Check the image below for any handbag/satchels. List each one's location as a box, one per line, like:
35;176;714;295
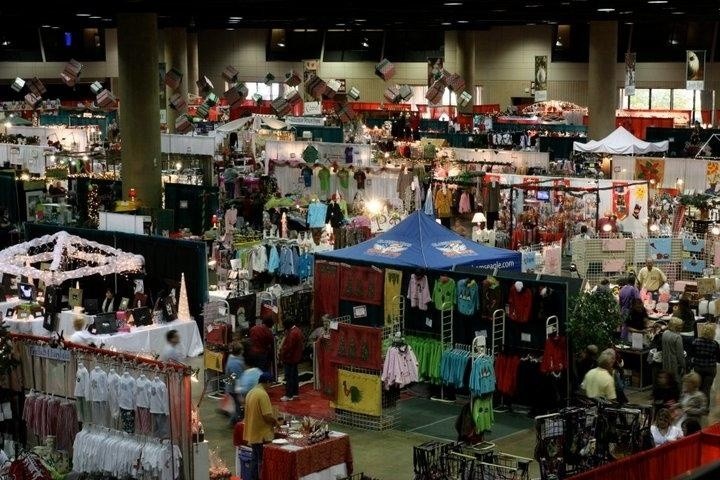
533;396;655;480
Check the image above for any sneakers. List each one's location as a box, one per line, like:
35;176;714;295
268;381;300;402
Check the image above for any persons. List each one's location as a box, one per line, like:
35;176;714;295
73;364;169;438
222;160;241;202
52;181;67;197
97;288;119;314
577;257;720;455
69;316;95;346
160;314;330;480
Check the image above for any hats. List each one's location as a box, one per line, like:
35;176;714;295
260;372;277;382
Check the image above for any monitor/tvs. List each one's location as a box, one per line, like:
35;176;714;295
536;190;550;201
353;305;368;319
17;282;37;301
